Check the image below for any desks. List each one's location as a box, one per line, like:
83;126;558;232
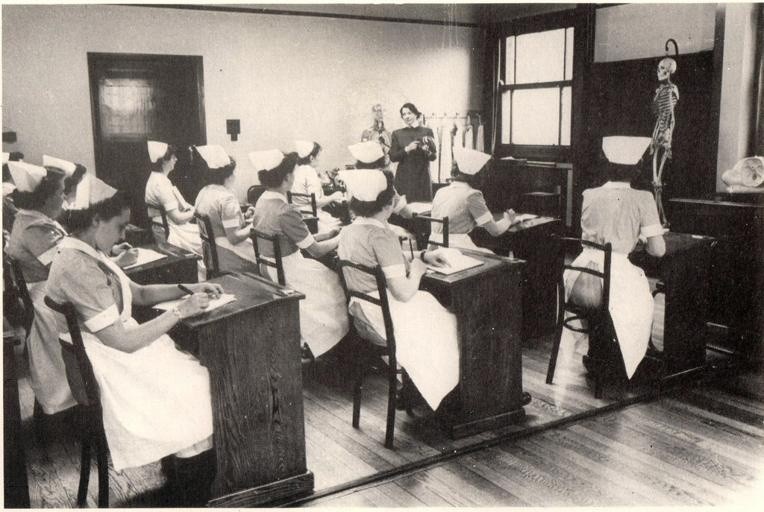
489;159;571;220
634;231;716;392
305;218;320;232
420;248;528;441
161;270;315;507
3;314;30;508
479;210;563;341
120;242;202;285
125;224;146;245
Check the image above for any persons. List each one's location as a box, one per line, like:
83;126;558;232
557;135;667;386
191;145;258;277
388;104;437;202
347;141;411;224
289;139;343;233
427;148;515;257
334;167;532;412
3;139;224;488
247;149;352;360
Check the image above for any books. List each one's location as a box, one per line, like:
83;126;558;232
423;247;485;275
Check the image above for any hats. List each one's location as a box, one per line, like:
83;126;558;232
247;149;285;172
2;152;10;166
348;139;385;164
42;154;77;179
452;146;492;176
195;144;232;170
296;140;315;159
146;139;169;164
74;174;119;211
7;160;48;194
601;134;653;166
337;168;390;202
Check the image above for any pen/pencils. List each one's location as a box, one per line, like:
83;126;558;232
123;245;131;250
409;239;414;258
178;285;194;295
505;208;509;214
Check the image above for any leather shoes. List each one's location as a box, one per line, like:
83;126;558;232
522;391;532;406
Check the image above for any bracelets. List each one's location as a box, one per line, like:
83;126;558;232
420;250;427;263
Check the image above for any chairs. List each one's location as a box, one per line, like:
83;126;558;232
193;213;227;279
3;251;35;357
247;227;328;388
546;231;617;399
522;185;562;218
287;191;317;218
43;294;110;508
142;201;169;242
333;251;424;450
412;212;449;249
